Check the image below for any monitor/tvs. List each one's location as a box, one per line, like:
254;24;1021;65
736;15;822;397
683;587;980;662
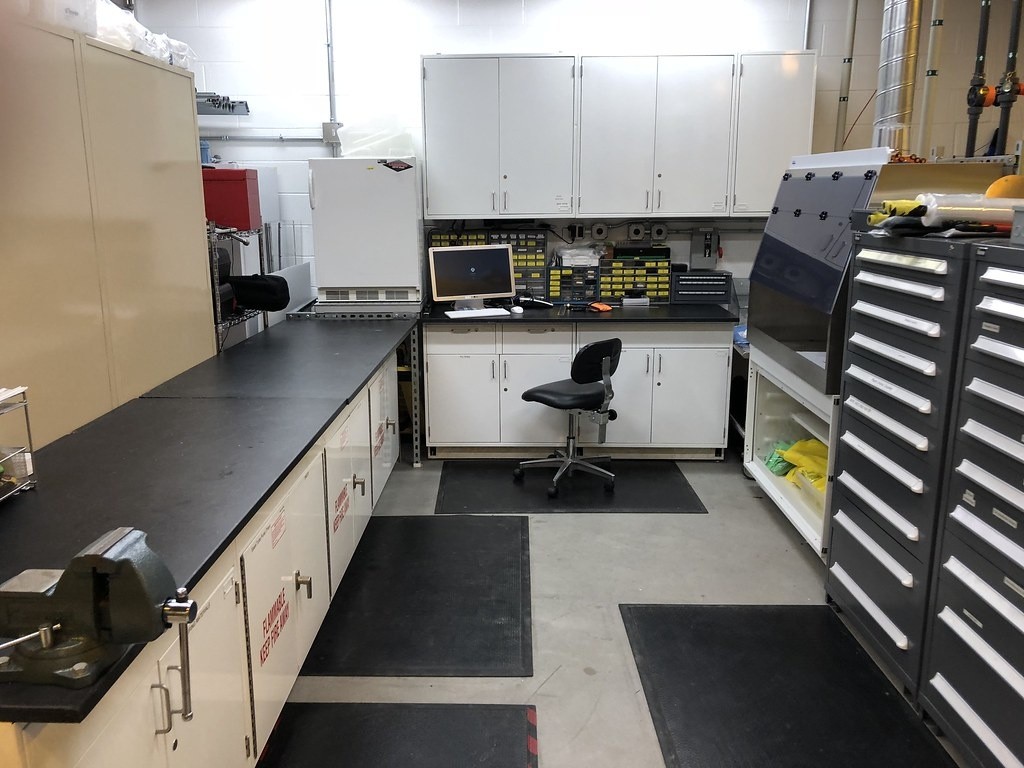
429;244;515;310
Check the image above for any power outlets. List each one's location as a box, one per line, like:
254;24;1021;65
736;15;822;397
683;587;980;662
569;223;585;240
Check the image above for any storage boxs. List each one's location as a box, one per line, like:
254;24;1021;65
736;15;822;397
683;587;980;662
202;168;262;231
796;471;826;519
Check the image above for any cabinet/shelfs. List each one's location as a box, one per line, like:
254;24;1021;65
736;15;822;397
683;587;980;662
0;10;218;452
420;50;819;219
1;349;400;768
743;344;841;567
422;322;576;459
823;231;1024;768
572;324;733;462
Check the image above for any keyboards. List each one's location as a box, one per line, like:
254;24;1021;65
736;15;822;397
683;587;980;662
445;308;511;318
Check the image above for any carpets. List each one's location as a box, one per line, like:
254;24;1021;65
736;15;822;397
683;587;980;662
435;459;709;514
617;604;960;768
255;702;538;768
298;516;534;677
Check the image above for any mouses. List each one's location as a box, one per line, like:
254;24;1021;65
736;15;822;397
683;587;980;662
511;306;523;314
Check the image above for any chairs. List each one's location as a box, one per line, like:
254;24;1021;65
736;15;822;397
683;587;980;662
512;338;623;498
